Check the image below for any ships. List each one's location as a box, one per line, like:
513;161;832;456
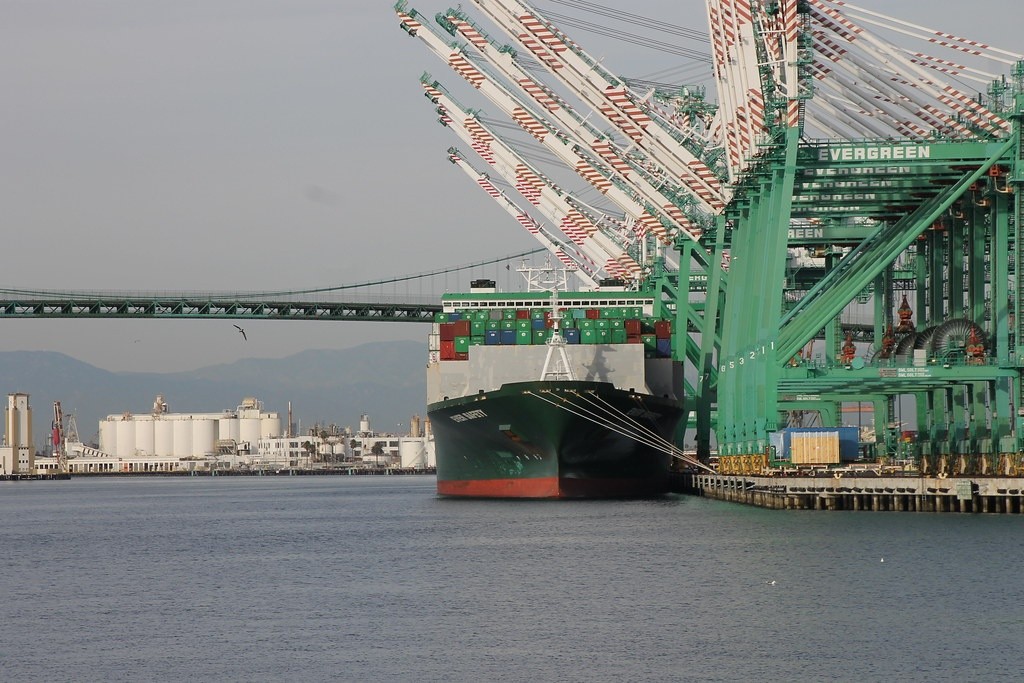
425;249;686;500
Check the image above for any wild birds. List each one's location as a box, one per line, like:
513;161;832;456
233;325;248;341
